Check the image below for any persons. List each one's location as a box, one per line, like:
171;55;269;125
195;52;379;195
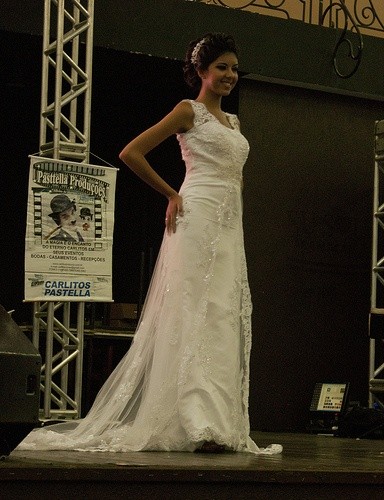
117;33;254;453
48;194;85;243
77;207;94;241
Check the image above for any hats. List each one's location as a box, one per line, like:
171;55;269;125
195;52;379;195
47;193;76;217
79;208;93;217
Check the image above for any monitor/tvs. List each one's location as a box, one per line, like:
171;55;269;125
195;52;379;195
306;380;350;416
